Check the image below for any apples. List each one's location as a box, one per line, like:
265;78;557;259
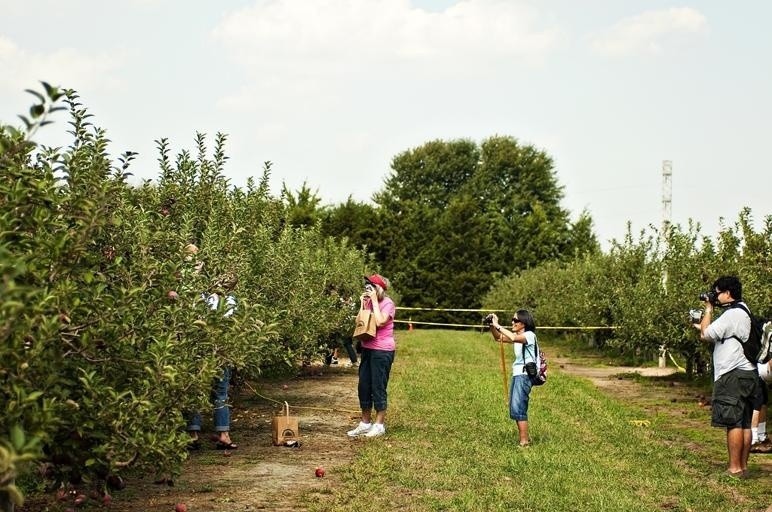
316;468;325;477
283;384;288;390
42;476;186;512
167;290;177;296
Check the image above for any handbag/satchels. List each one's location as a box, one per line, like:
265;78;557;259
272;400;299;447
352;294;377;341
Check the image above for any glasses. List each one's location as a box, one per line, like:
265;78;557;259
511;317;520;324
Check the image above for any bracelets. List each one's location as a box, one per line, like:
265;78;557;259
495;325;502;331
703;307;712;313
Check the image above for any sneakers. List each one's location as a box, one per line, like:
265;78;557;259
331;357;360;369
721;467;749;479
365;423;385;438
750;439;772;454
347;421;371;438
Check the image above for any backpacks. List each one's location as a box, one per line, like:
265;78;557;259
532;351;547;386
719;305;772;365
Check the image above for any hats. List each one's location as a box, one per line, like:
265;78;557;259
365;274;388;291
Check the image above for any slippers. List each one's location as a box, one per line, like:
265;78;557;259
216;441;238;449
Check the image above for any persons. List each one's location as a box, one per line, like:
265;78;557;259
346;272;397;437
167;241;238;449
748;315;771;454
486;309;540;449
700;275;760;476
303;280;360;368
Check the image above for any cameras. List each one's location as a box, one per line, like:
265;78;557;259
700;292;717;304
482;314;492;324
363;283;371;291
